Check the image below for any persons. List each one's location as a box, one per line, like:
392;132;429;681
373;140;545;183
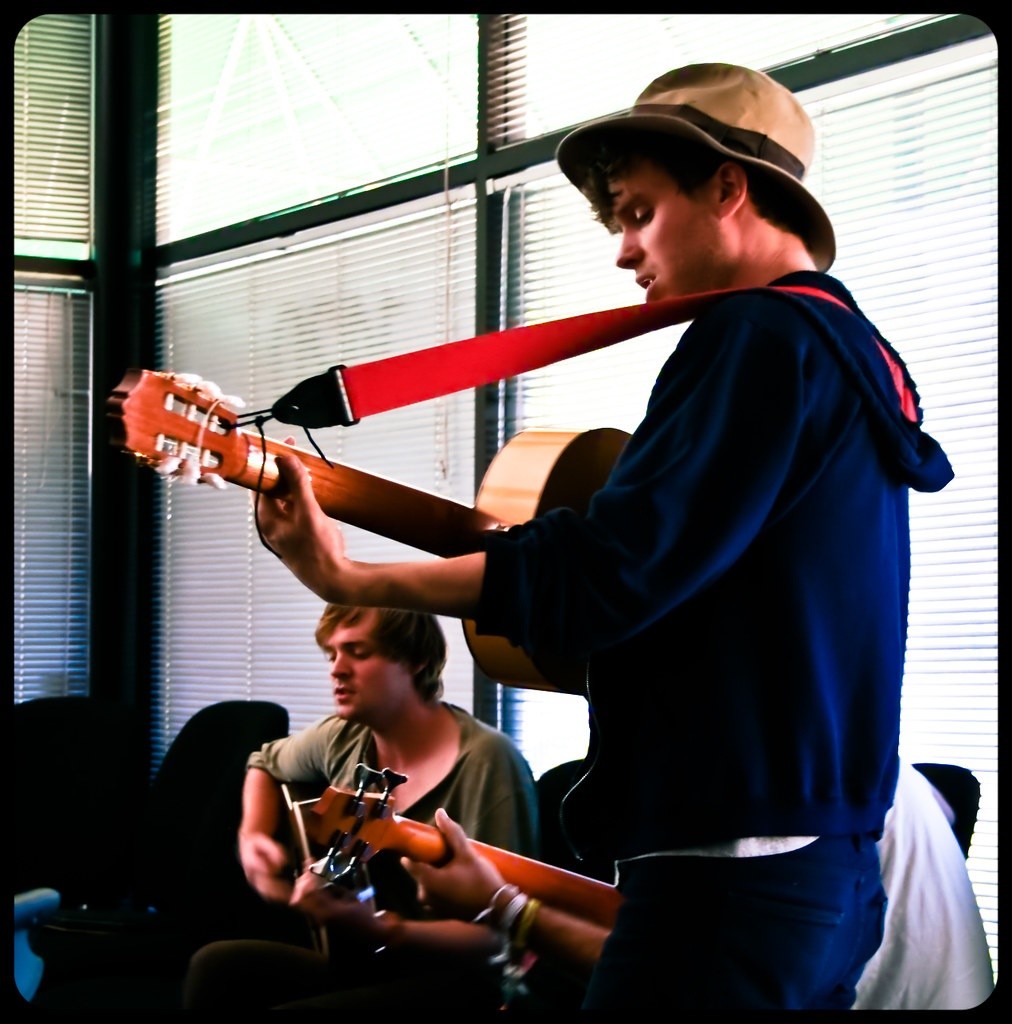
253;63;956;1024
182;603;538;1024
401;752;994;1024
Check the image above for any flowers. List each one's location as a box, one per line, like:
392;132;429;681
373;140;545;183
47;701;288;937
14;694;133;907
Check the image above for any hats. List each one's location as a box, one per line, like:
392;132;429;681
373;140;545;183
556;63;836;273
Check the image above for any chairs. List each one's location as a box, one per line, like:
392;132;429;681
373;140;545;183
913;761;981;861
536;758;585;876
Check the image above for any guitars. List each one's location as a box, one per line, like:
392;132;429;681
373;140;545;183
312;764;623;943
282;760;405;923
122;368;636;688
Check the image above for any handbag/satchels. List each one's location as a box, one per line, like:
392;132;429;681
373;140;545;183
28;908;161;979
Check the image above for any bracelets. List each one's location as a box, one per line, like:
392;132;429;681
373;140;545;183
485;882;542;941
